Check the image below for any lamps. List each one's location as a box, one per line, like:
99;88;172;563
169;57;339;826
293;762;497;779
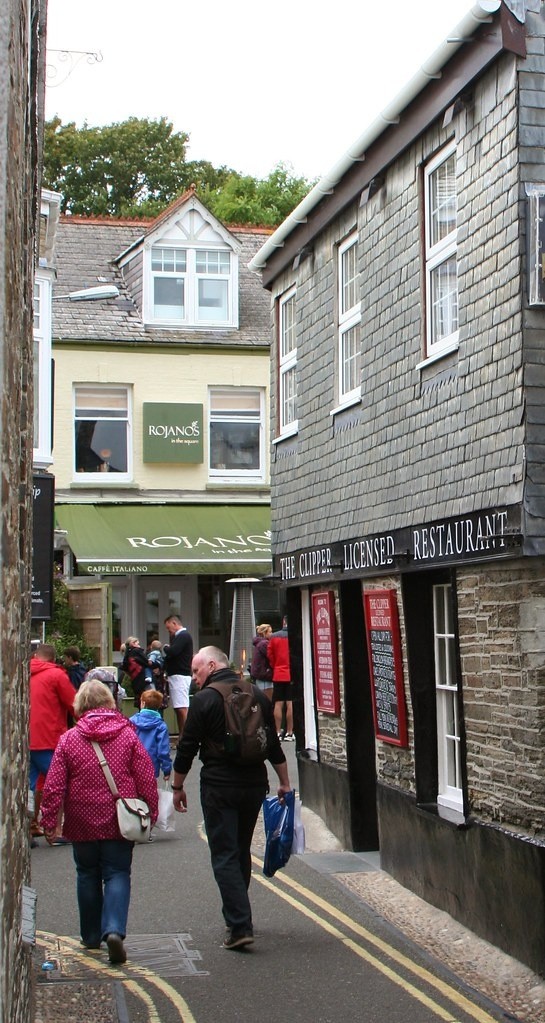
52;285;119;301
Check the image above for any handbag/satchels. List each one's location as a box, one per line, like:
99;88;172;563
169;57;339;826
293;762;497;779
113;796;152;843
261;787;306;878
155;780;177;833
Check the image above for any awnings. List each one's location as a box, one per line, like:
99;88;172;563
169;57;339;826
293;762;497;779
54;504;274;575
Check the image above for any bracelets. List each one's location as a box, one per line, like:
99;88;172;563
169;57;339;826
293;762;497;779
171;784;183;790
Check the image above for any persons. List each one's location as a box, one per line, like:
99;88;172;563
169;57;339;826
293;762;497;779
119;614;193;842
30;644;86;846
171;646;292;949
40;680;159;963
250;615;295;741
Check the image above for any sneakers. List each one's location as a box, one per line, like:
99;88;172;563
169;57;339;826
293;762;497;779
277;729;283;741
222;926;254;948
283;732;296;742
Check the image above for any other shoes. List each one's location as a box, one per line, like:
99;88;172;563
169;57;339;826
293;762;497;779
169;741;178;750
32;829;45;836
51;837;70;846
31;842;40;849
106;933;127;964
79;936;100;949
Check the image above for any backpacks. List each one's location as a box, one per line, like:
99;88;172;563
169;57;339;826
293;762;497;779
198;680;271;767
